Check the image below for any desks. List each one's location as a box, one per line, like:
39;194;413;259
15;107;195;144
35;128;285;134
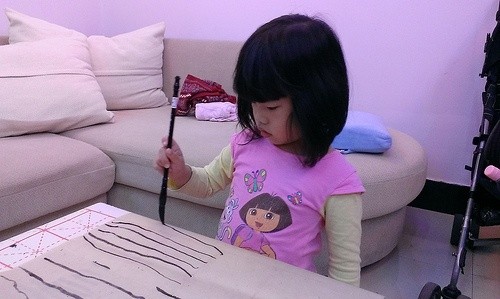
0;202;385;299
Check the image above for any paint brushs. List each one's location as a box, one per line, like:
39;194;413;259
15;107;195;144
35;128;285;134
157;73;182;228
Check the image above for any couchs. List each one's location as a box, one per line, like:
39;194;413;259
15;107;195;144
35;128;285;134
0;35;115;136
0;36;428;275
5;9;169;110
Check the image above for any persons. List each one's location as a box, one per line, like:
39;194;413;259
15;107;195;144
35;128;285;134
152;13;366;288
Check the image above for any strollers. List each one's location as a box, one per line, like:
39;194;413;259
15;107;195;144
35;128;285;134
417;0;500;299
